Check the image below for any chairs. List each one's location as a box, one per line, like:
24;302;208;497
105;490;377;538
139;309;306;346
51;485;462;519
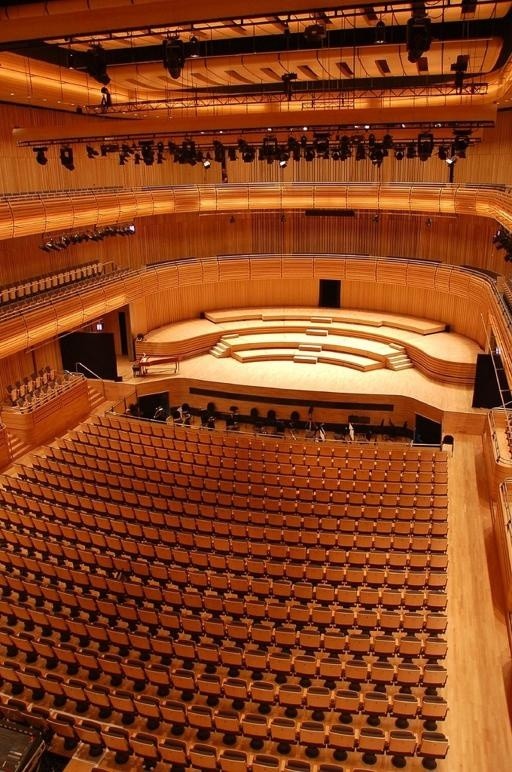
6;366;73;414
1;402;451;772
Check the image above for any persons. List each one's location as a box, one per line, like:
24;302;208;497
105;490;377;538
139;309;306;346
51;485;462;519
154;395;169;420
172;403;241;431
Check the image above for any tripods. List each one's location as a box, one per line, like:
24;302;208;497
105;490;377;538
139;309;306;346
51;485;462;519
304;413;319;433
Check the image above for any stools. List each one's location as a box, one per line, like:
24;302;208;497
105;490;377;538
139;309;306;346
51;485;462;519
133;366;141;378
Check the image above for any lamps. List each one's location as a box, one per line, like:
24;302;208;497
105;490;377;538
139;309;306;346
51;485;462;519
374;12;387;43
86;40;110;86
161;36;185;79
31;129;474;171
188;35;200;58
406;9;432;62
